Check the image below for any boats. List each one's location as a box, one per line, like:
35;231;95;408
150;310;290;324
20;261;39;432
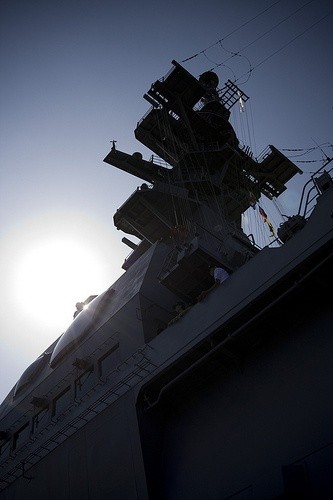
0;40;331;500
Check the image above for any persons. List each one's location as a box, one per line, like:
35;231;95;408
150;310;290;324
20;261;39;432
197;263;229;299
